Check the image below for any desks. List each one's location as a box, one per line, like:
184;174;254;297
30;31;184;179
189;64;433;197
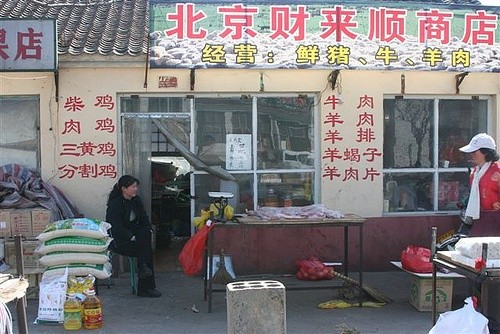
203;213;367;313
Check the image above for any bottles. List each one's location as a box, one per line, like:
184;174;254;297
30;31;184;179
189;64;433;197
82;289;102;329
64;292;81;330
266;189;277;206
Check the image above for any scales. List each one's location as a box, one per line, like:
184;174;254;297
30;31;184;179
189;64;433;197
208;192;235;225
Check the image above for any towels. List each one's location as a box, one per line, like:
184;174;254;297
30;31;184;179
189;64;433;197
465;160;492;220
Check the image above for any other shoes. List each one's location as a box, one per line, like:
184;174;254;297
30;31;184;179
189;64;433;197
137;288;161;297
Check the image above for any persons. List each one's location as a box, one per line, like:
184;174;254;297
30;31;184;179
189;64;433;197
460;132;500;237
106;175;162;297
440;135;463;166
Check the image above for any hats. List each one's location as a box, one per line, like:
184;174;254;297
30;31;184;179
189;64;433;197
460;133;496;153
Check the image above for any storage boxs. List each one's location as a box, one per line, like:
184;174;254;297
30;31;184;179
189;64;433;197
0;207;54;299
409;274;453;312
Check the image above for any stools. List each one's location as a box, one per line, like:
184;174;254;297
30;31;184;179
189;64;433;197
108;252;138;296
